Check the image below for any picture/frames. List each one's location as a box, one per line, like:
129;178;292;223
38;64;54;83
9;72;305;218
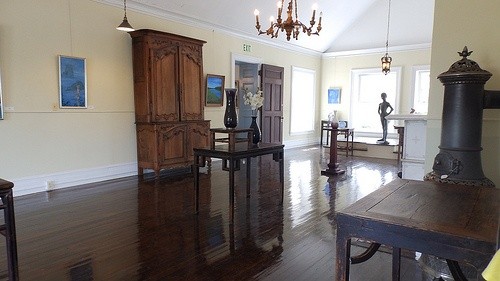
205;74;225;106
327;88;341;105
58;55;87;109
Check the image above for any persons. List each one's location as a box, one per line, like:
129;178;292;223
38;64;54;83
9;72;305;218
378;93;393;141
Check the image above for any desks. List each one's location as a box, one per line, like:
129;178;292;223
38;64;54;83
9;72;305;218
335;178;500;281
384;114;426;180
0;178;19;281
320;120;354;157
192;127;284;225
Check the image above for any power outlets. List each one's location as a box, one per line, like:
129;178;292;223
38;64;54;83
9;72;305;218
46;181;54;192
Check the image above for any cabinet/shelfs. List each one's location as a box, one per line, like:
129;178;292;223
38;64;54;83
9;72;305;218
127;29;212;177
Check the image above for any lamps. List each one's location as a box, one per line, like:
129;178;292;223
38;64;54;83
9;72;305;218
381;0;392;75
254;0;323;42
116;0;134;31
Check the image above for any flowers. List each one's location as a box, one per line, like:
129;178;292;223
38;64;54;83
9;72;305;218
241;87;264;116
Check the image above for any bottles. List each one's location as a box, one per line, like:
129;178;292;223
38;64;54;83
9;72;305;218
224;88;238;129
330;110;338;130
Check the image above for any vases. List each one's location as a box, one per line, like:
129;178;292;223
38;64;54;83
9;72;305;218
248;117;261;145
224;88;238;129
